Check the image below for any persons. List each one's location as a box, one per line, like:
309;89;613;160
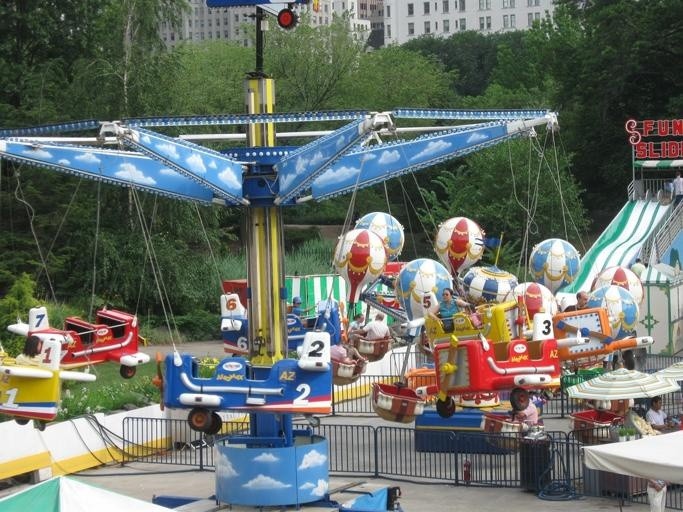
418;287;473;348
563;291;591;313
646;397;683;432
289;298;320;328
506;397;539;433
672;171;683;208
663;179;674;200
632;258;650;279
15;337;44;365
329;312;390;367
646;479;670;512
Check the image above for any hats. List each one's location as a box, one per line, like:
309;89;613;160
292;296;301;303
